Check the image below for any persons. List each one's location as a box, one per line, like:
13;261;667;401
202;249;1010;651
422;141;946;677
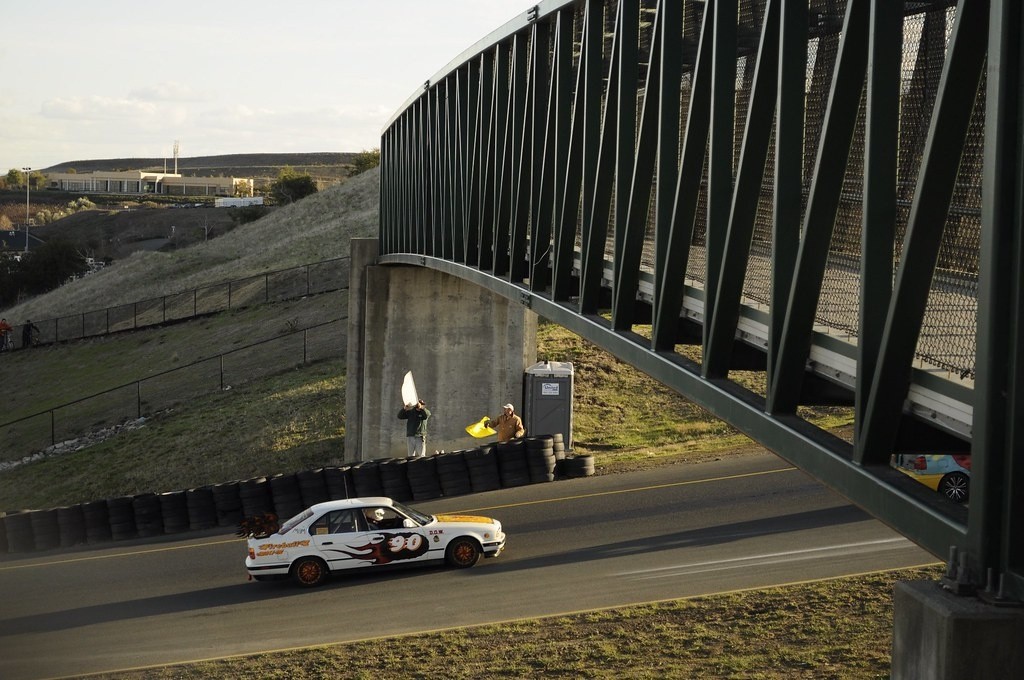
398;398;432;459
484;403;525;445
367;508;399;530
0;319;40;350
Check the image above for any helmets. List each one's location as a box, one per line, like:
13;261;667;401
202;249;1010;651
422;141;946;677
367;507;384;521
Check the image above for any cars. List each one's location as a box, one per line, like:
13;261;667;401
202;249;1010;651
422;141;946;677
893;453;974;503
244;496;507;591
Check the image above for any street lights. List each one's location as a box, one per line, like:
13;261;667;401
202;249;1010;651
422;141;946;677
23;167;32;253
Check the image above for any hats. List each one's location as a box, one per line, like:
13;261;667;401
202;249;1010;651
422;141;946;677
502;404;514;412
419;399;426;406
